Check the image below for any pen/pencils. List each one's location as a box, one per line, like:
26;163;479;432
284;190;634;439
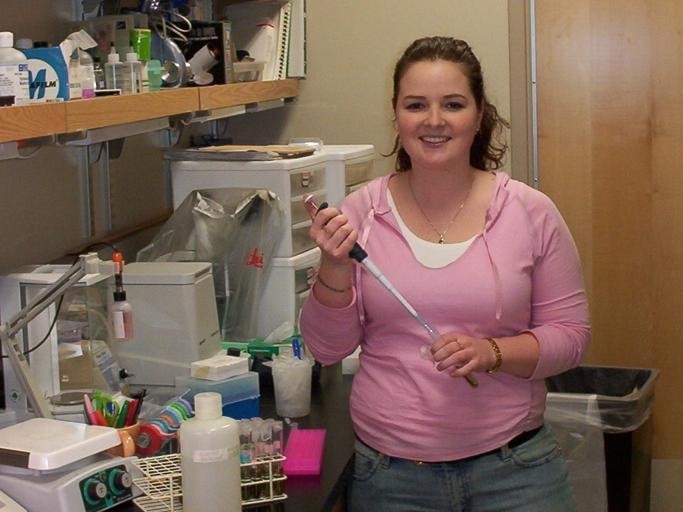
84;390;145;428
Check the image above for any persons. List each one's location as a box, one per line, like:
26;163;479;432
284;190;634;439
302;38;590;512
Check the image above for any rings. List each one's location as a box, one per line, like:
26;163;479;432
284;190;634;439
456;341;462;350
323;227;331;236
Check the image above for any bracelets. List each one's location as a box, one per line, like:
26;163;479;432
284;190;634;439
316;274;352;292
485;336;503;375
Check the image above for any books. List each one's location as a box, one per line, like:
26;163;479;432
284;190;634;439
225;3;291;81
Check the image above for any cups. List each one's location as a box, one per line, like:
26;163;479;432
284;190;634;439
272;360;315;419
103;424;139;459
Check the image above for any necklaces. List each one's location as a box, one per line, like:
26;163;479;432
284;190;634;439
408;172;475;248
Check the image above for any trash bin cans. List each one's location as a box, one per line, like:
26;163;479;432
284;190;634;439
544;366;659;512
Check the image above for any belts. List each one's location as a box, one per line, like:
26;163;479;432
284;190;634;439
354;425;543;464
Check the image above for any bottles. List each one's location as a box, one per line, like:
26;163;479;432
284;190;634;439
104;41;142;93
427;328;480;387
178;391;284;511
1;30;30;103
110;290;133;340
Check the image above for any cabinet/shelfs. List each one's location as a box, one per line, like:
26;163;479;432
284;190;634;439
170;144;376;337
